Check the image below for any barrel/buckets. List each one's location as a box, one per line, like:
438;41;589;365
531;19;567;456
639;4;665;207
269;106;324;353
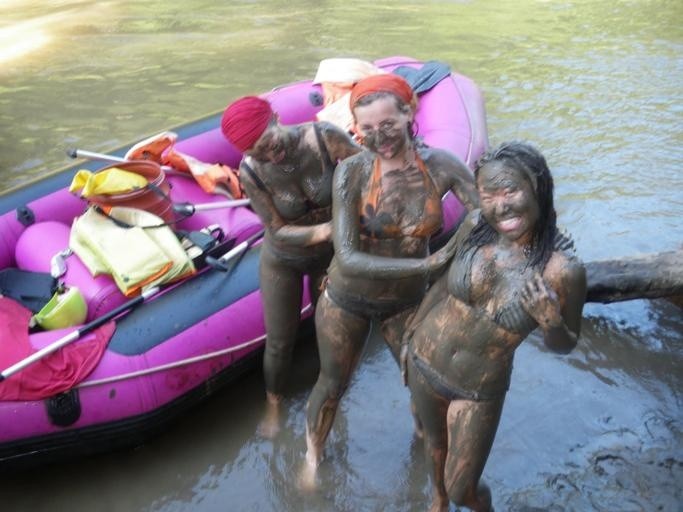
89;160;176;234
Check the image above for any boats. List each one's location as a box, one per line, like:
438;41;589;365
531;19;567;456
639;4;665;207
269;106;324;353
0;50;493;470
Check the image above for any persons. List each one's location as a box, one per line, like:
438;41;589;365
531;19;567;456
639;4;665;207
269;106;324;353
291;72;576;495
395;138;588;511
220;95;429;444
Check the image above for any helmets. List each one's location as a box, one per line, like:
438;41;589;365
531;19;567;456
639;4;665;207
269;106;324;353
36;287;87;329
